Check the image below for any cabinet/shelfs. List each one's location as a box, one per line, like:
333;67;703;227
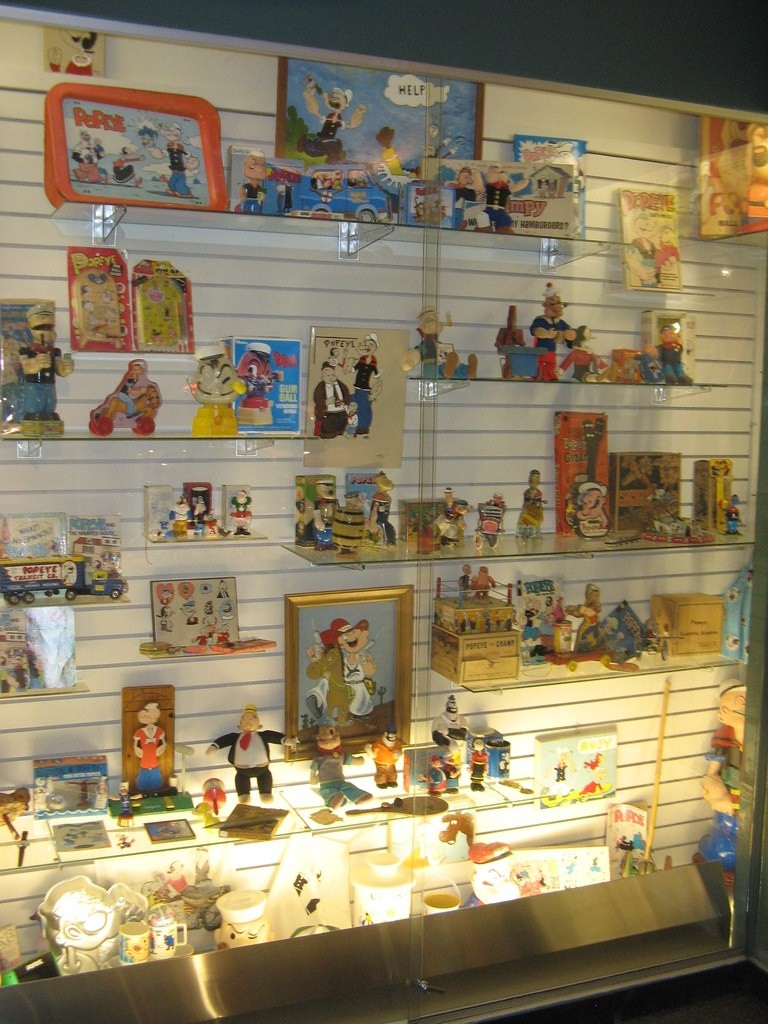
0;194;768;1023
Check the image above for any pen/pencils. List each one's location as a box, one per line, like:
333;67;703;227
202;814;284;828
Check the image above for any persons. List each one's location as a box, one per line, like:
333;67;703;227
20;303;75;421
701;679;747;870
648;326;693;384
438;487;469;544
212;889;275;951
110;781;142;816
476;162;529;234
309;716;372;809
567;584;602;651
314;471;397;559
169;490;254;536
404;306;479;379
726;495;739;532
522;468;543;536
206;705;297;803
529;285;611;382
427;695;488;797
239;150;267;213
458;564;496;601
364;724;407;789
464;841;520;907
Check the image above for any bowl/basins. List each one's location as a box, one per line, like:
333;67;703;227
215;888;266;922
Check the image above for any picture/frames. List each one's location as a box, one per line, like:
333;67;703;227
280;583;416;764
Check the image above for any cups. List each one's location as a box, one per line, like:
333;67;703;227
421;874;462;915
117;921;151;966
149;909;188;960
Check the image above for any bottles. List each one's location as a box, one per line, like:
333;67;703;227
351;853;413;926
